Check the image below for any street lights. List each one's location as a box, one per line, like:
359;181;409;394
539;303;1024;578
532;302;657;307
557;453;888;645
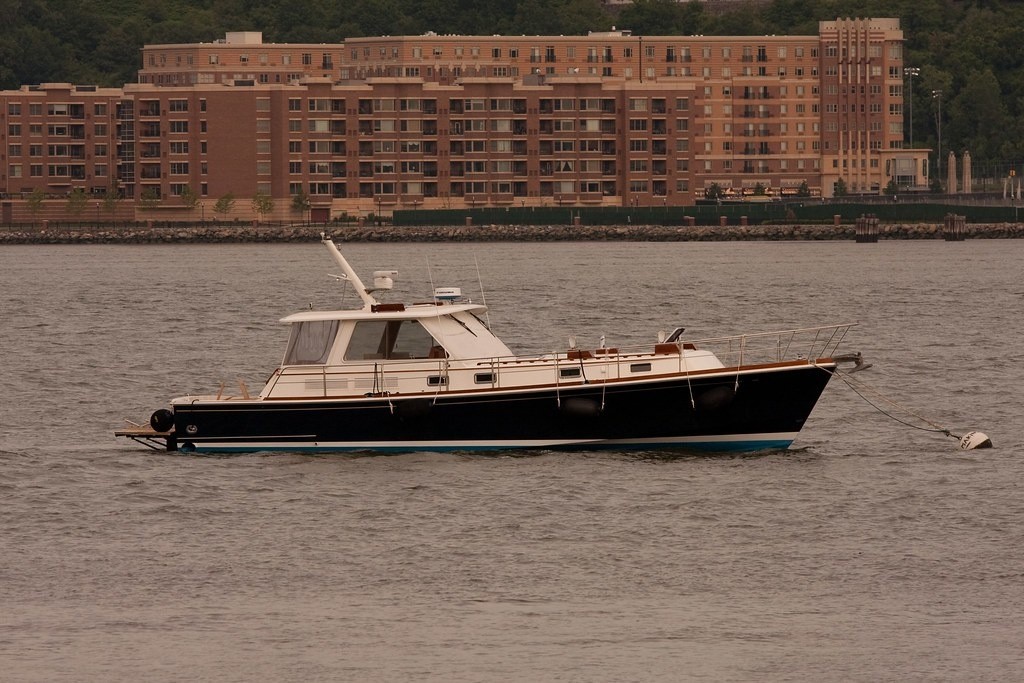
522;199;525;207
471;195;476;208
903;65;920;150
559;195;562;206
1011;194;1015;207
630;198;634;207
663;198;667;205
200;201;205;227
635;195;639;206
413;199;418;210
95;202;100;232
306;200;311;227
893;195;897;203
932;89;942;185
377;196;382;228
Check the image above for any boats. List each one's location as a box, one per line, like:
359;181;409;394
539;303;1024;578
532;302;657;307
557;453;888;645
111;229;877;456
